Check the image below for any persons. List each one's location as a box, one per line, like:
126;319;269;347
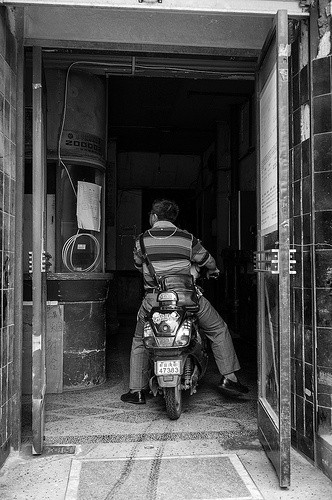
121;200;250;405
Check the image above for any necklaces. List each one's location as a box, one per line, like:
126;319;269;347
147;225;177;240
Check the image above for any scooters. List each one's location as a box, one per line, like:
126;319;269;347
143;291;209;419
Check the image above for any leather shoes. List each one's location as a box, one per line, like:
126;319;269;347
121;390;146;403
218;375;249;394
